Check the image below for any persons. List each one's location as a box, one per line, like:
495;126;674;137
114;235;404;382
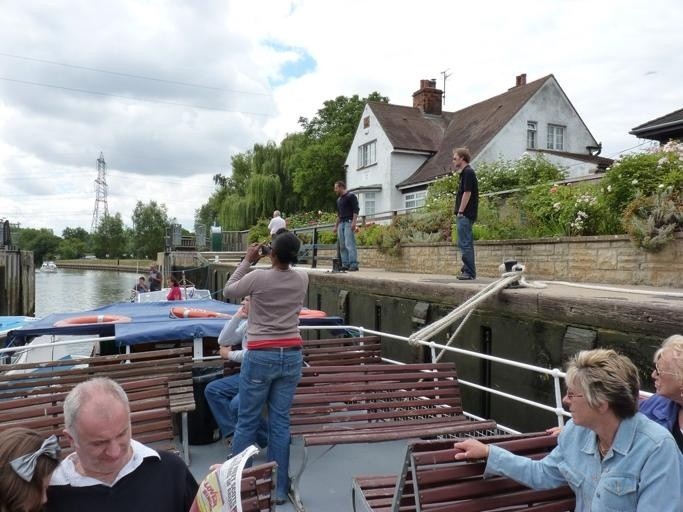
222;228;309;505
0;426;62;511
453;347;683;512
149;263;162;292
452;147;479;280
268;210;286;242
203;295;270;448
167;275;181;301
333;181;360;271
133;277;148;293
41;376;223;511
546;333;683;455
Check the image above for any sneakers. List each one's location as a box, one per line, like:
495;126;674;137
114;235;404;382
348;266;360;271
338;266;348;270
458;276;474;280
456;274;462;278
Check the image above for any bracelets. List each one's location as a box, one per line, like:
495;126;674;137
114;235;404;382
458;212;463;214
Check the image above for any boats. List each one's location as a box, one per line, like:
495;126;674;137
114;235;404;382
0;299;572;512
40;261;59;274
133;288;215;302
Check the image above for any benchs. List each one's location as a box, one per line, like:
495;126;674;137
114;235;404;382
224;337;381;379
288;363;496;512
0;347;196;469
0;377;179;464
411;435;576;512
350;431;548;511
197;460;279;512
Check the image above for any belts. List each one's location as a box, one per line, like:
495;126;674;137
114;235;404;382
247;345;303;353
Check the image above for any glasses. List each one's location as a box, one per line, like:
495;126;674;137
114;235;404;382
653;361;683;378
564;388;592;400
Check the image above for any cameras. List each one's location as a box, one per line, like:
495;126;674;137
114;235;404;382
260;241;272;256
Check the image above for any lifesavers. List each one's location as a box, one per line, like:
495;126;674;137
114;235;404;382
170;305;233;320
54;315;132;327
296;308;326;318
